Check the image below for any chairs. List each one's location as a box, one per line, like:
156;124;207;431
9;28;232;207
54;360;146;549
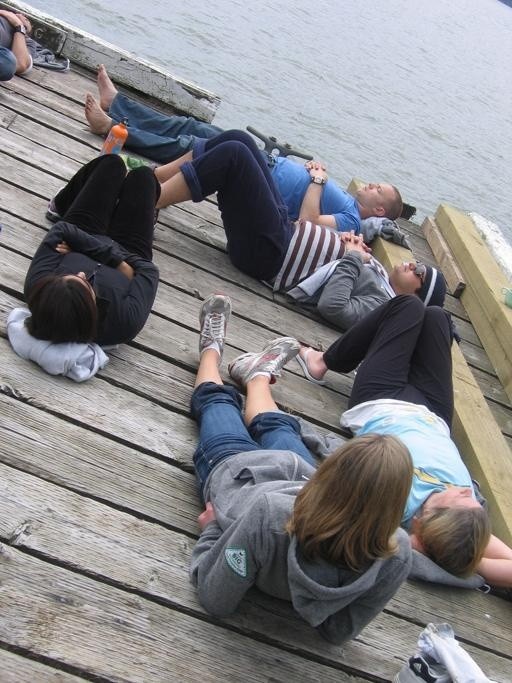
390;650;452;683
45;182;70;223
117;153;158;173
198;293;232;368
228;337;301;386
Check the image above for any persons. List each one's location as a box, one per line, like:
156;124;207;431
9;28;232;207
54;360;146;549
24;156;165;347
76;61;405;228
178;288;426;653
293;295;512;595
0;9;35;82
120;128;446;329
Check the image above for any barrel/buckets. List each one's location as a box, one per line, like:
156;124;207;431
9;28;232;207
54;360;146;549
295;347;326;386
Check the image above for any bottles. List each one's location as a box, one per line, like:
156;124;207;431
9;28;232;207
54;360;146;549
415;264;447;308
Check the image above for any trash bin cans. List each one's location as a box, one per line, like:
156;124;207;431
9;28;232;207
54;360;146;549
13;23;26;33
310;175;326;185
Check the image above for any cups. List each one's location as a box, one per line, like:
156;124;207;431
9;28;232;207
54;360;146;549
413;260;425;286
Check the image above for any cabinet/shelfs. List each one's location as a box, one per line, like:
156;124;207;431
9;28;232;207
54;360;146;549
100;117;130;158
